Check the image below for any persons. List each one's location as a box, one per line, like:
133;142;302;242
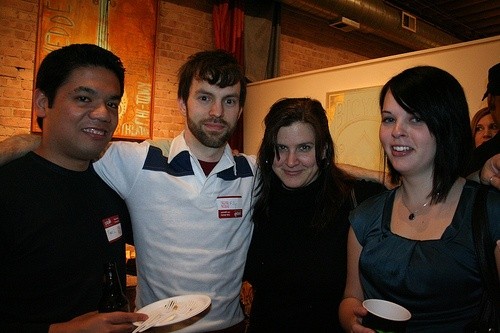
463;107;500;173
337;65;500;333
0;38;149;333
466;61;500;189
237;97;500;333
1;46;403;333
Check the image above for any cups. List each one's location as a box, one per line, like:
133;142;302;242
361;299;412;333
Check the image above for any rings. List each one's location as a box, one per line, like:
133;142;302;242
488;175;497;184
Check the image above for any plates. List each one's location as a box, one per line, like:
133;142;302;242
131;294;212;327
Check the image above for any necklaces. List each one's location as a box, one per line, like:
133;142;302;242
401;182;439;221
